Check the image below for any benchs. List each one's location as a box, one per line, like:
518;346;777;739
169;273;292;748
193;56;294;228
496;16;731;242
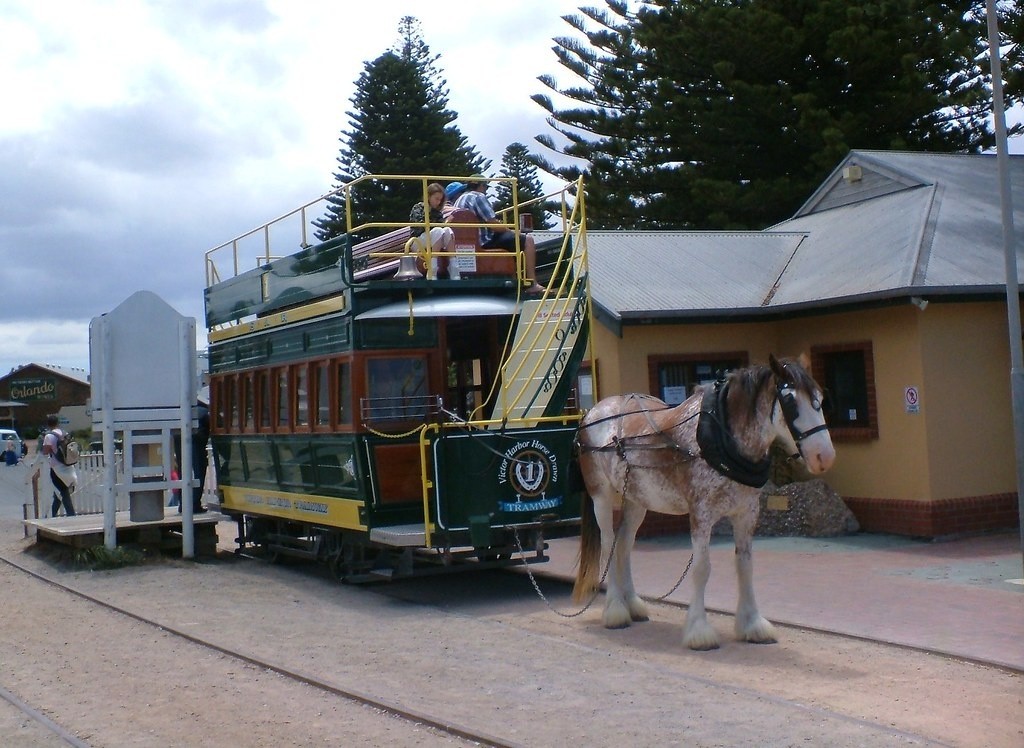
352;227;412;280
444;209;517;276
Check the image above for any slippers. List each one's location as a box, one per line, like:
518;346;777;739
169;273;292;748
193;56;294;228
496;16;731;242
527;287;555;295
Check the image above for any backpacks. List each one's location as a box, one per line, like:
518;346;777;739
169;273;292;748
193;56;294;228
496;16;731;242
44;429;78;466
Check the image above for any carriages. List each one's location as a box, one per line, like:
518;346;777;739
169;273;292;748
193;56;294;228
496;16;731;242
204;171;839;651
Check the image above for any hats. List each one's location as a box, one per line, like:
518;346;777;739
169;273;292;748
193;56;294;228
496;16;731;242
8;436;13;439
444;182;468;200
468;174;492;187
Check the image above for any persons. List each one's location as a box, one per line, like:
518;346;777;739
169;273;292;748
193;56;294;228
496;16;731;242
445;173;554;293
170;397;211;515
409;183;460;280
6;413;77;518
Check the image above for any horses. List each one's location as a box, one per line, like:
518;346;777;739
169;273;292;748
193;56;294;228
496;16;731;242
569;351;836;650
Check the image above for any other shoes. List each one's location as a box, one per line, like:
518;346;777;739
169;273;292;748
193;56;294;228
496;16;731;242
447;264;461;280
178;503;208;513
426;267;438;280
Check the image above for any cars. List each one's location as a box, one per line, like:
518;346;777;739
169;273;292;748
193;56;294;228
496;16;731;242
0;430;22;462
